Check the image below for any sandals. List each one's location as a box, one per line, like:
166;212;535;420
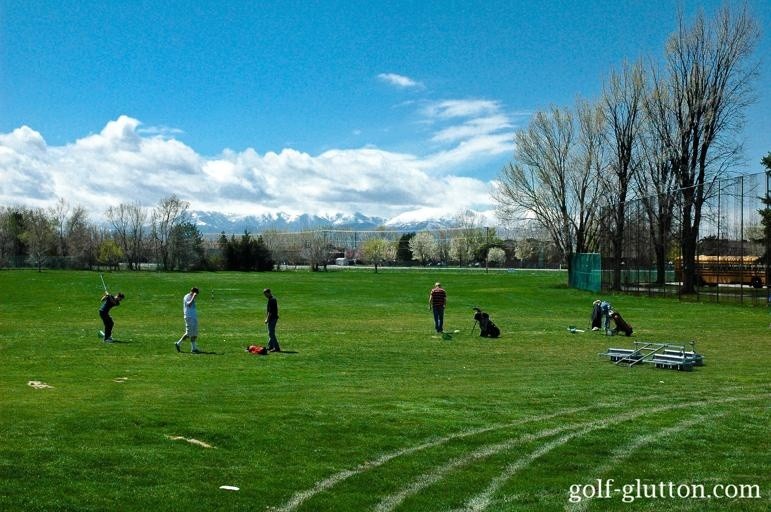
674;254;767;289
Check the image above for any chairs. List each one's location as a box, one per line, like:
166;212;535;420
175;343;180;352
191;350;199;353
267;345;281;352
104;337;115;343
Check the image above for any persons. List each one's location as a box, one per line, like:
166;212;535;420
174;288;202;354
97;290;126;343
262;288;281;353
429;282;447;333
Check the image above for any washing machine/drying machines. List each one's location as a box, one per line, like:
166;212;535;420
471;308;481;333
101;273;107;294
